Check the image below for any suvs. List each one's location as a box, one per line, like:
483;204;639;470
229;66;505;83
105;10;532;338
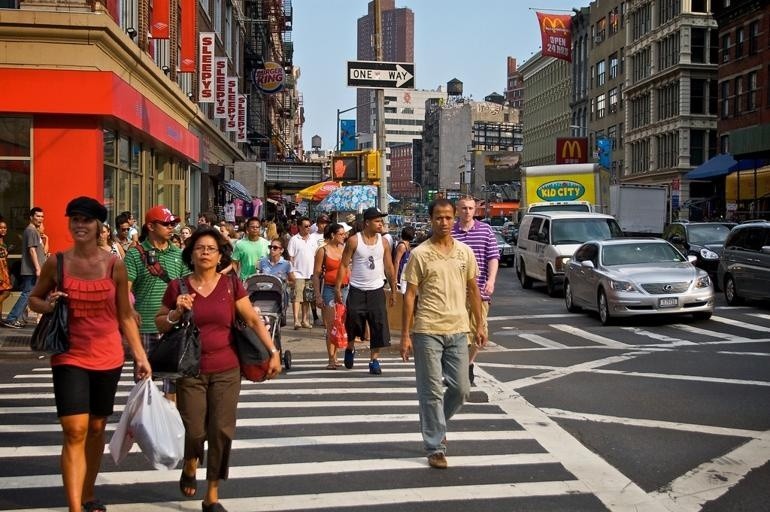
513;212;624;298
666;219;770;304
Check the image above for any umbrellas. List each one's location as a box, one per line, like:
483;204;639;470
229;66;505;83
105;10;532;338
300;180;338;202
317;185;399;211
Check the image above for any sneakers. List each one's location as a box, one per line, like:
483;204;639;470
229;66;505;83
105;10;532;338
428;436;448;469
368;358;381;375
4;319;23;329
442;377;448;388
294;318;324;331
468;362;474;387
327;347;354;370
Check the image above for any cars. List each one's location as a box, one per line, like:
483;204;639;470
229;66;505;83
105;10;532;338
563;238;715;325
388;214;518;268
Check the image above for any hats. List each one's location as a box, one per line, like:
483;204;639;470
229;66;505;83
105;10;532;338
144;205;181;225
364;208;389;221
64;195;107;223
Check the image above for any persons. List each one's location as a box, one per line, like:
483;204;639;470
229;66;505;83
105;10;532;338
124;205;190;401
393;224;417;324
98;211;357;327
28;197;152;512
382;221;393;263
334;208;397;375
399;198;487;470
0;207;48;329
453;196;499;388
154;226;280;511
313;223;350;369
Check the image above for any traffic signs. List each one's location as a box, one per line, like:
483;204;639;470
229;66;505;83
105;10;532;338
348;61;415;88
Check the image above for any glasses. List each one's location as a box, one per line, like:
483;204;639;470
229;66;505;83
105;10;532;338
120;227;130;232
269;245;282;249
193;245;219;254
249;224;260;229
153;220;176;227
304;224;310;228
368;256;376;270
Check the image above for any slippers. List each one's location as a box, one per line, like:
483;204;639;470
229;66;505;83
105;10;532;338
201;501;226;512
180;457;198;498
81;498;106;512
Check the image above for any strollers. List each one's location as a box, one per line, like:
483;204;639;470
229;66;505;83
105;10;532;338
241;273;291;369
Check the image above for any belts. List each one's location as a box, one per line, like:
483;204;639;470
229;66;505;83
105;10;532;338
327;284;349;289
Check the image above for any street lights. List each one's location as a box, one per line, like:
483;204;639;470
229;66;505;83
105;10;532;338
409;179;422;211
568;124;614;186
337;98;391;150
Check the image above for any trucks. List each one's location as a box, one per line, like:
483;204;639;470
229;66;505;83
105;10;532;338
519;167;614;211
609;184;670;237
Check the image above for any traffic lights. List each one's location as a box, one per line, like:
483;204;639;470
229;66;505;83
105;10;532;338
333;156;358;180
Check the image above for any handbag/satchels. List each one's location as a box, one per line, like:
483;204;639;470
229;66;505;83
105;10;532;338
147;316;204;385
232;319;272;383
304;275;324;303
29;296;70;358
384;289;414;332
